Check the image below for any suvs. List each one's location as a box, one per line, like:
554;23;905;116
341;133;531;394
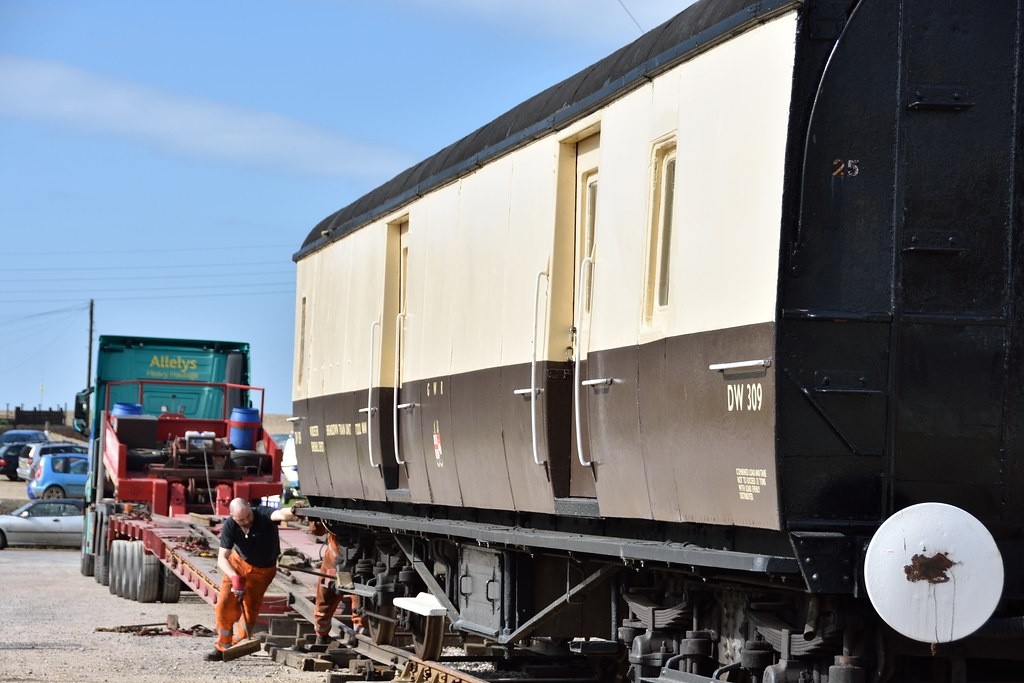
0;441;42;481
15;440;88;481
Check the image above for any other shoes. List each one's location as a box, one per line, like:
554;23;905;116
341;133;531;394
315;634;327;645
204;648;223;661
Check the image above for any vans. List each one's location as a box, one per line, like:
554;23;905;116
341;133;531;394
0;429;49;442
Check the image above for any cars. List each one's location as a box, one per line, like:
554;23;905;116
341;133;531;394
271;434;292;458
27;453;89;500
0;498;88;550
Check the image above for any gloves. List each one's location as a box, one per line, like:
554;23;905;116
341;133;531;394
231;577;244;597
291;501;306;520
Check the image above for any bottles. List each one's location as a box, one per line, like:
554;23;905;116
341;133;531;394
261;495;281;509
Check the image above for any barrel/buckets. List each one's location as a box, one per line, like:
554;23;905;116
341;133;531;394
230;406;261;450
110;403;142;428
185;431;216;449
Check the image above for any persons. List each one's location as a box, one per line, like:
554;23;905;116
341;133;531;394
203;497;307;662
309;520;369;643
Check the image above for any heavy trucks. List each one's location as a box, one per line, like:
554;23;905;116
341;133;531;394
73;335;340;634
